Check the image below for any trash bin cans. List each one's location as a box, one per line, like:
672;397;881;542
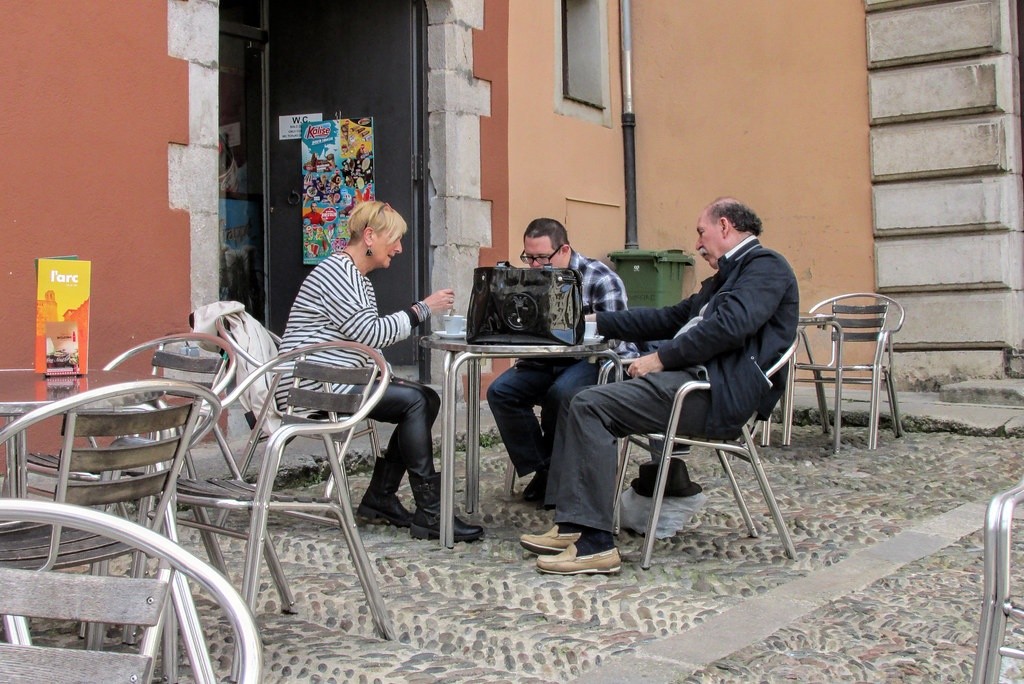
607;249;695;308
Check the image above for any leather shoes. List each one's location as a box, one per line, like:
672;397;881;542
410;510;484;543
357;497;413;528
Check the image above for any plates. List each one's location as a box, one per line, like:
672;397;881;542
434;331;467;339
583;336;605;344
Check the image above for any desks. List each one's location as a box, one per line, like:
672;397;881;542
0;368;176;650
419;335;623;549
761;311;844;447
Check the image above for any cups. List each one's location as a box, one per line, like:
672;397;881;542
442;314;467;334
585;321;597;337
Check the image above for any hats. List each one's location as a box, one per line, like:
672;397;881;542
632;458;702;498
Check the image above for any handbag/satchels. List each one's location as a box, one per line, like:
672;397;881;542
466;261;586;346
619;486;706;539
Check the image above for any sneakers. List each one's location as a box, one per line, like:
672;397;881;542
520;525;581;556
536;544;620;575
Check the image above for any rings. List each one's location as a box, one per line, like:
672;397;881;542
449;300;453;303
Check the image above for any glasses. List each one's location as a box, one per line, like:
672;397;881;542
521;245;561;265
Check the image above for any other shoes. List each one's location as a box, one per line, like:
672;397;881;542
536;501;555;511
522;471;549;501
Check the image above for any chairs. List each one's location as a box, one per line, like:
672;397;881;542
0;299;391;684
596;329;796;570
972;476;1024;684
782;293;906;449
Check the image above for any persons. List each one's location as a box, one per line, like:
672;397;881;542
272;203;484;543
487;219;643;496
519;196;798;575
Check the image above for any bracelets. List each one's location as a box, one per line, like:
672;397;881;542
414;301;432;321
